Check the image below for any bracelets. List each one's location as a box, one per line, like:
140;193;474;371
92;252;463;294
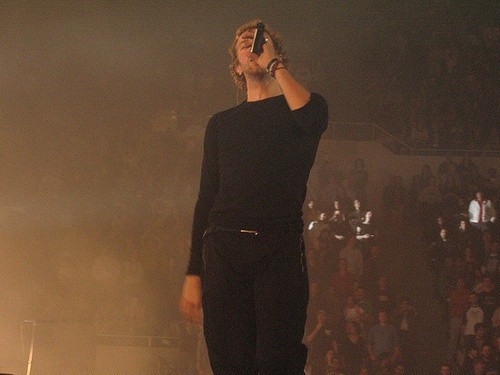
265;57;286;77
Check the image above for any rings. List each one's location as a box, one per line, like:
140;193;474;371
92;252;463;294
264;38;269;43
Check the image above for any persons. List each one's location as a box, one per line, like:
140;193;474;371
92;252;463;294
179;18;329;375
304;160;500;375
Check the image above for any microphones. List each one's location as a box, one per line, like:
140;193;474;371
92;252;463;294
251;23;265;55
